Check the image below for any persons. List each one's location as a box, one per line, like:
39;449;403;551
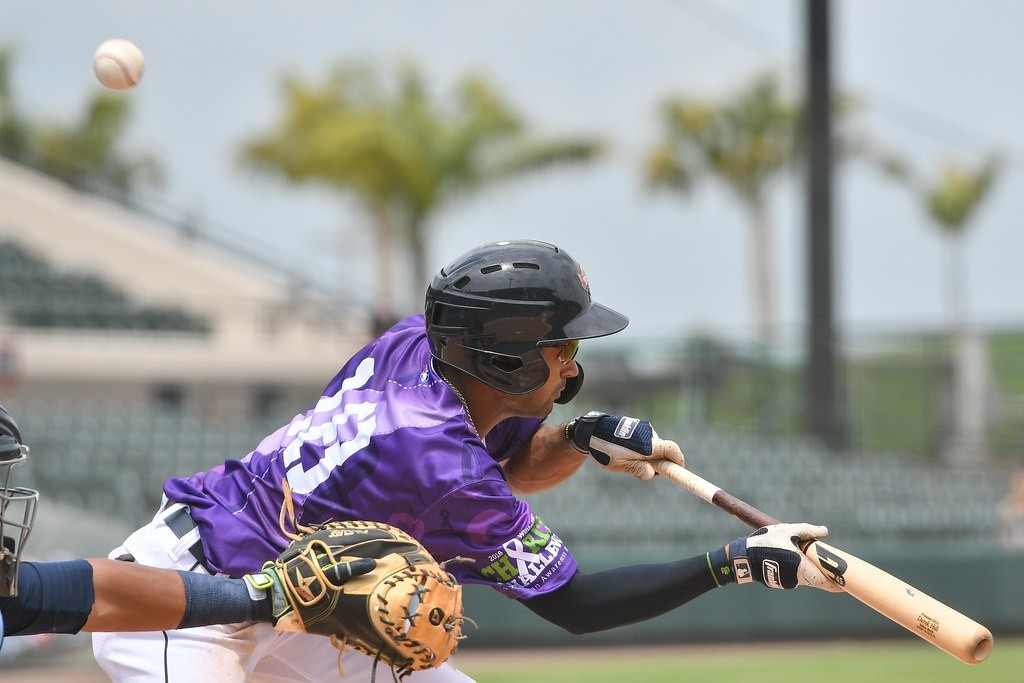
91;239;845;683
0;408;478;683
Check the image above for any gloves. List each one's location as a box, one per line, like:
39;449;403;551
728;523;846;593
565;410;684;481
242;550;375;627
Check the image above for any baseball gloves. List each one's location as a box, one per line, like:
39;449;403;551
239;475;481;683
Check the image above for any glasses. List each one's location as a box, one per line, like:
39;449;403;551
539;340;580;363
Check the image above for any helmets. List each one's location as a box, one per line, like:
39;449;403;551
425;239;628;394
1;406;38;597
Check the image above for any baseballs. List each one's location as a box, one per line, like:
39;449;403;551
93;37;146;91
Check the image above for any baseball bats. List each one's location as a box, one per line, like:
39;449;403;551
652;458;998;667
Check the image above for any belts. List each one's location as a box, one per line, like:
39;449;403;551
165;500;239;580
